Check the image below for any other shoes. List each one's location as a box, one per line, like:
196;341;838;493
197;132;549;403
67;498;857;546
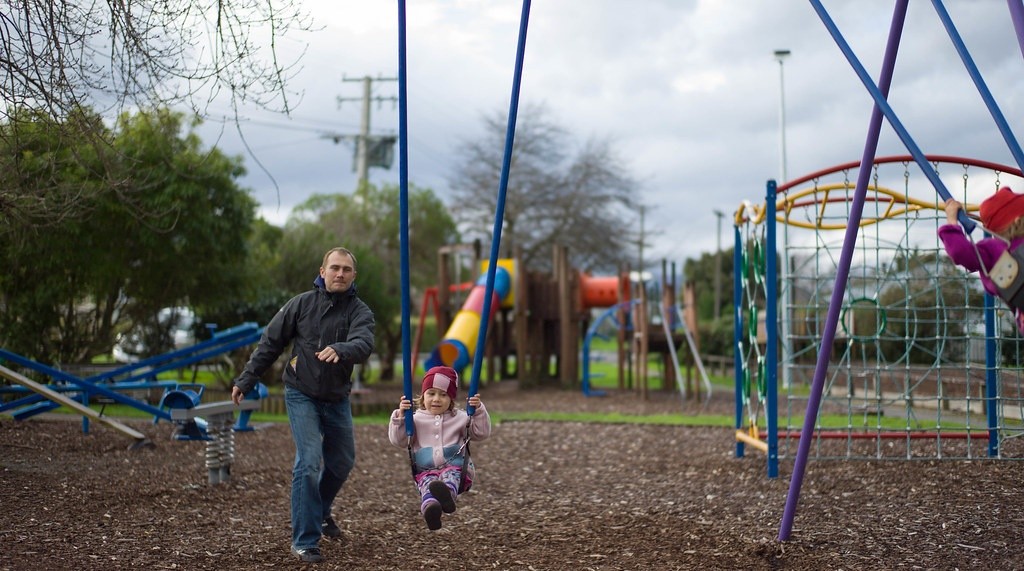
431;481;456;514
424;502;442;530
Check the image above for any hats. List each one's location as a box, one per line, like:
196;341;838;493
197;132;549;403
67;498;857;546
421;365;460;398
980;187;1024;232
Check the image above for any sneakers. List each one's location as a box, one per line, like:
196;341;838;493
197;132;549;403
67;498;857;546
321;518;341;537
292;545;321;562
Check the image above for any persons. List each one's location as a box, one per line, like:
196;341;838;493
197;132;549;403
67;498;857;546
232;246;376;562
387;371;492;531
937;194;1024;337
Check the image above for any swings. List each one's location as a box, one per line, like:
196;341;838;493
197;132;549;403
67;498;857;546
809;0;1024;335
396;0;534;500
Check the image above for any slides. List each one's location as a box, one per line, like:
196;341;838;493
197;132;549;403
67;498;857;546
422;264;513;374
